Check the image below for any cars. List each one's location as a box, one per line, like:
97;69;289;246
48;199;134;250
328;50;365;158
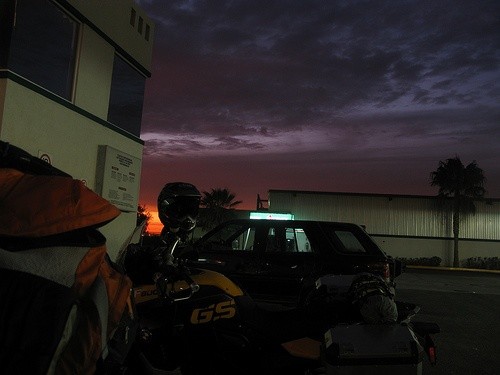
188;217;393;308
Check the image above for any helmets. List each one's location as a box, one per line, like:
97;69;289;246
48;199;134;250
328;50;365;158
157;182;201;233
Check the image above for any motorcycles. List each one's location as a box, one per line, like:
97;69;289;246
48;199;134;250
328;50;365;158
112;216;439;373
